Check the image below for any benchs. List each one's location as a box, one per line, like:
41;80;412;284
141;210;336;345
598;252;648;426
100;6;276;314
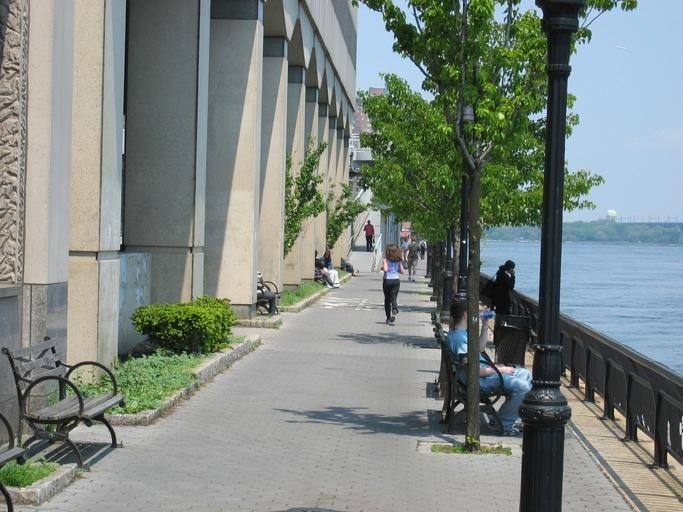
257;280;279;315
432;312;505;435
0;335;125;512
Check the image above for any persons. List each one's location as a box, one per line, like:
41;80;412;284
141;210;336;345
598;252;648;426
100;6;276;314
380;245;404;325
363;220;374;252
255;270;277;315
490;261;516;349
314;249;359;290
399;234;426;283
447;301;531;435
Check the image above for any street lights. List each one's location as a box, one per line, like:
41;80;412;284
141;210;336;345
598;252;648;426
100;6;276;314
514;0;587;512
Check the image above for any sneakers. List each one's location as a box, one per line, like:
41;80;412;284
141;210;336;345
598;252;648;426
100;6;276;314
489;420;523;435
333;284;340;288
391;309;397;321
386;319;391;324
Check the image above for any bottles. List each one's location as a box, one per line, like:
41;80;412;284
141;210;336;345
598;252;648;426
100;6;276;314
478;310;497;320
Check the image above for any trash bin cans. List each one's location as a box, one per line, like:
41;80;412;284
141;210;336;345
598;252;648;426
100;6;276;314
493;314;532;368
